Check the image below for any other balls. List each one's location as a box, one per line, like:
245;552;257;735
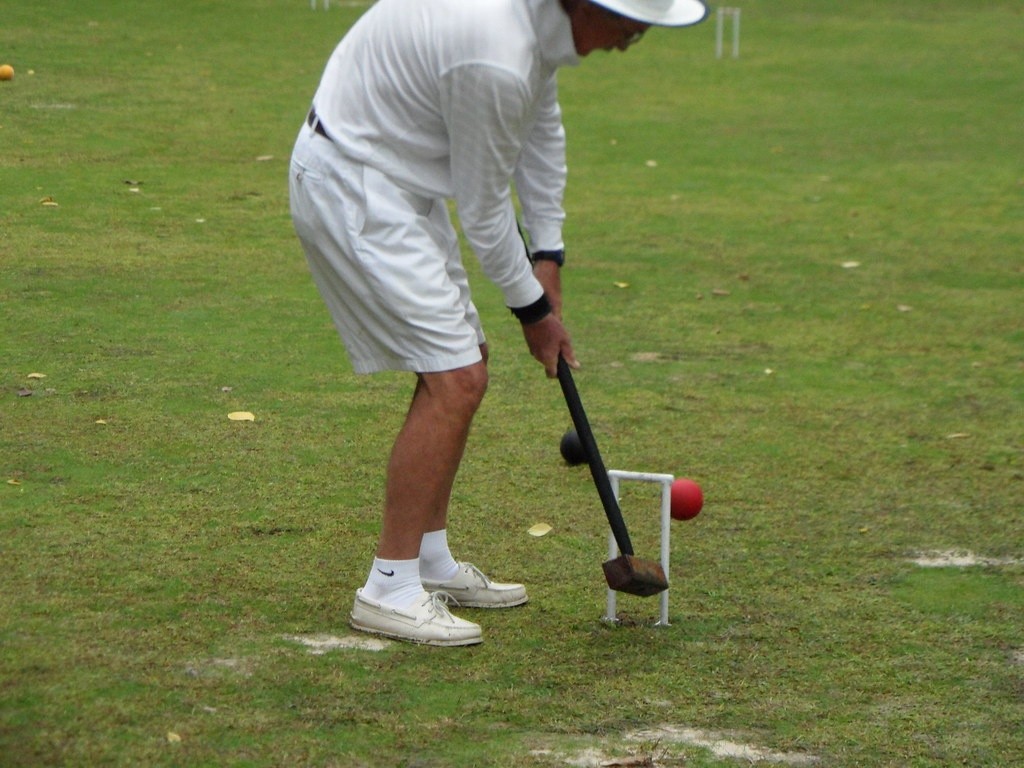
0;64;14;80
559;429;597;466
660;477;704;520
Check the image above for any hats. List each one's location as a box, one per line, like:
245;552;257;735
590;0;706;26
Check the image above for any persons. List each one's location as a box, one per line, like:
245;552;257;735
286;0;711;649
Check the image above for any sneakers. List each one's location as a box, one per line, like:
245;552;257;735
349;588;483;647
420;561;528;608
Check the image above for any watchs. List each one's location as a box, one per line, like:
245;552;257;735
530;250;568;267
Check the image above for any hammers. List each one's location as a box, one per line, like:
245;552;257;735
516;221;668;598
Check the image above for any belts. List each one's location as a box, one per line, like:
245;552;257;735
308;107;332;143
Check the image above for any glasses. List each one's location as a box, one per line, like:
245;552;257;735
591;4;644;44
